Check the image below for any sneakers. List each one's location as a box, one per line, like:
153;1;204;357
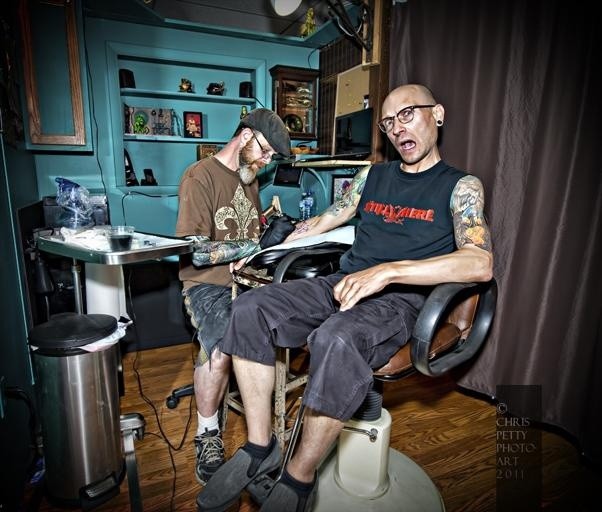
260;467;319;511
197;434;283;511
194;427;227;487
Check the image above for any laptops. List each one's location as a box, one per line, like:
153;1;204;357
298;107;374;162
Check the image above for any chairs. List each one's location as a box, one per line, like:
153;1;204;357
244;244;498;511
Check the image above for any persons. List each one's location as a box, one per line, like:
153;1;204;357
196;85;493;511
177;108;319;488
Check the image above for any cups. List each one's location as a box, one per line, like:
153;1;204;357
103;225;135;252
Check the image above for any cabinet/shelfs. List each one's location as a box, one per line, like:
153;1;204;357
107;41;266;186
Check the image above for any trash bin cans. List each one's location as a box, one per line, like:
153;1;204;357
28;312;127;510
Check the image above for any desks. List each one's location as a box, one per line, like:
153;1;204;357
35;227;193;316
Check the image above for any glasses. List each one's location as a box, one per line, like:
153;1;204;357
247;128;279;159
377;104;437;132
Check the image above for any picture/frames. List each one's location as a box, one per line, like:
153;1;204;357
330;173;357;206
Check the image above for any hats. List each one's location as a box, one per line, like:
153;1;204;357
237;108;291;157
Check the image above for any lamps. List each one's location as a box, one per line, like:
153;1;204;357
268;0;305;21
